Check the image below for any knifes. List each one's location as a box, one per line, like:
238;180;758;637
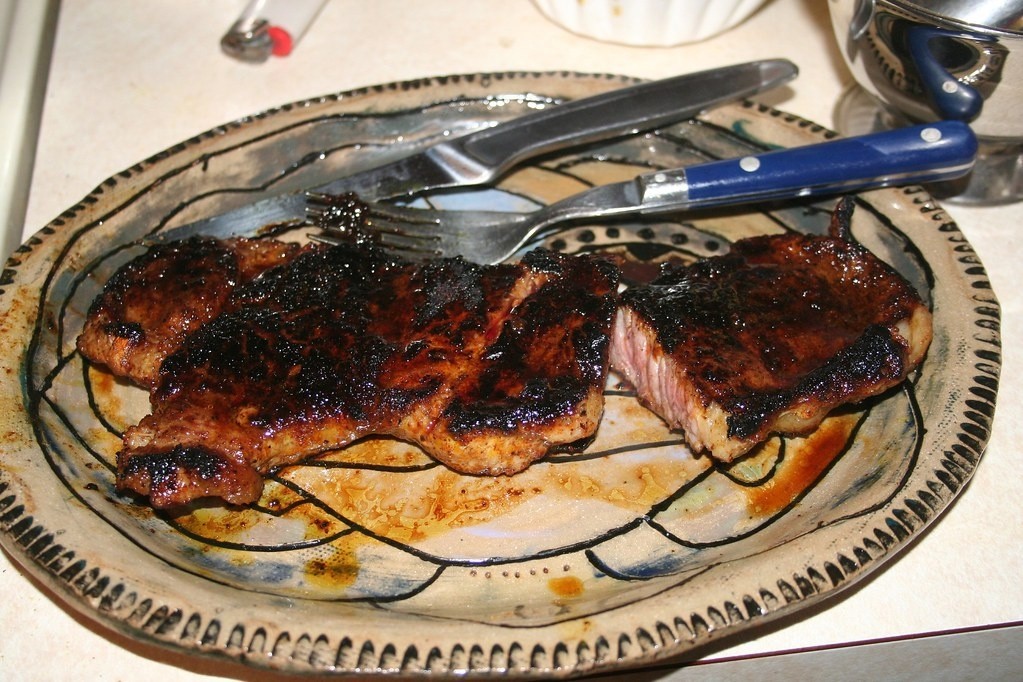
141;53;798;244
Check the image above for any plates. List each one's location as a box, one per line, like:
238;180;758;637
0;69;1003;682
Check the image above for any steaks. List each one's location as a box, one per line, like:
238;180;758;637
75;233;932;512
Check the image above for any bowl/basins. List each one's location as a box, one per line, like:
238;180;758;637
533;0;767;49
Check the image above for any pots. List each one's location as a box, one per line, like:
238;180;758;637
831;0;1023;208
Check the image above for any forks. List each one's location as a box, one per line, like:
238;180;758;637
304;116;978;266
